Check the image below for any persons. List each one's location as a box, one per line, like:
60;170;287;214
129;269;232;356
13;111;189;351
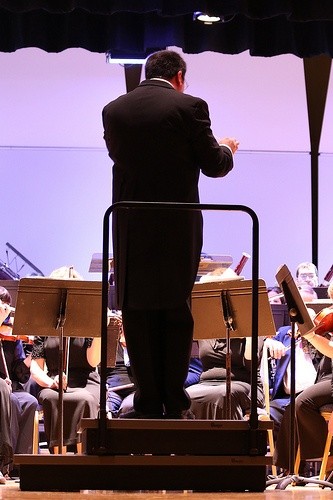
102;50;240;419
0;261;333;485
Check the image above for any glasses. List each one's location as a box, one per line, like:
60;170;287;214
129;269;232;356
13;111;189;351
184;78;188;90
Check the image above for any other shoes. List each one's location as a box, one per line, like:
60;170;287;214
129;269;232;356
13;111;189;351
266;471;288;479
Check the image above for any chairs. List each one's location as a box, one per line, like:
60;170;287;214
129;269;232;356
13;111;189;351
8;344;333;489
